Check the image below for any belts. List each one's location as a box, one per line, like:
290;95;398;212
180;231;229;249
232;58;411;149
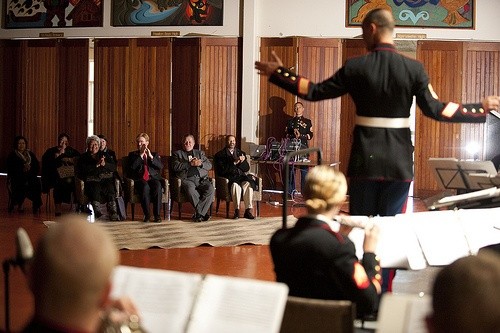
354;115;409;128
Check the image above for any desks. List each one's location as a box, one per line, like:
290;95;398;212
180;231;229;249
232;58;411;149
252;159;342;207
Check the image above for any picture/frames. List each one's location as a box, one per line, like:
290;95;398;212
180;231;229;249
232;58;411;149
4;0;105;30
346;0;476;31
111;0;224;27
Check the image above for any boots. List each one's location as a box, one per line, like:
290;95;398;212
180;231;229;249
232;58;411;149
107;200;120;220
92;201;104;218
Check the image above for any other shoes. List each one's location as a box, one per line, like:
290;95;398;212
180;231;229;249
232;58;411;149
203;213;211;221
155;216;162;222
55;211;61;216
234;213;239;219
244;213;255;219
144;216;151;222
76;204;82;211
194;212;202;222
19;209;25;213
288;194;295;199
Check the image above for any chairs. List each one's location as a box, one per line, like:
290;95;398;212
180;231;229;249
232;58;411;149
278;296;355;333
1;155;263;222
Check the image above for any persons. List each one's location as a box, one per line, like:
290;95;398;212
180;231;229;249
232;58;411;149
171;135;214;222
78;134;124;221
255;9;500;216
14;214;148;333
269;165;382;320
42;134;82;217
7;137;43;217
213;135;255;219
424;254;500;333
124;133;163;223
283;102;314;201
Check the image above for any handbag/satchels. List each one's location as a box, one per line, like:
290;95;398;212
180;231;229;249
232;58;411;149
57;161;74;178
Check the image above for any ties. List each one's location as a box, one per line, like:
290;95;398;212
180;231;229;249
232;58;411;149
143;153;148;172
